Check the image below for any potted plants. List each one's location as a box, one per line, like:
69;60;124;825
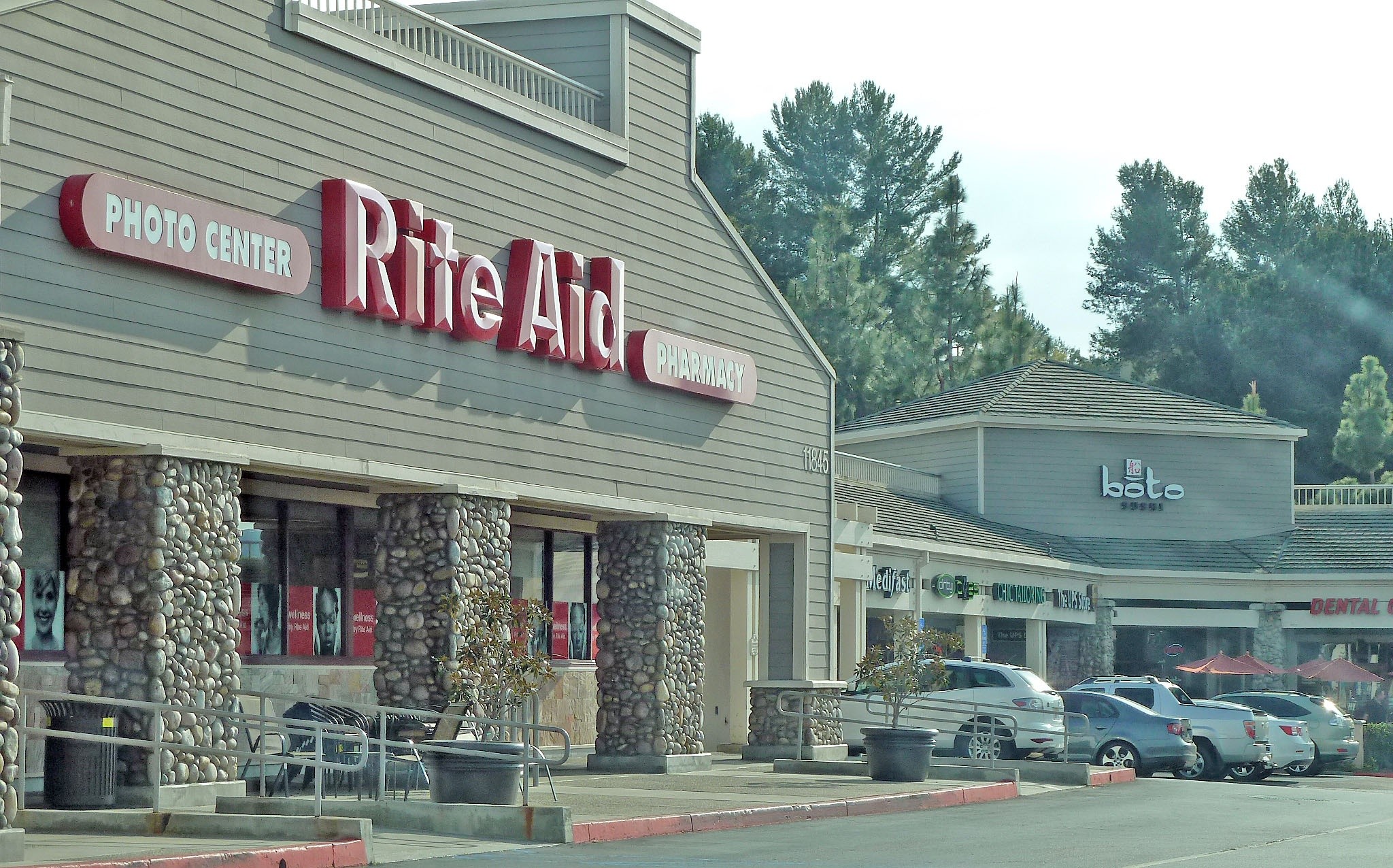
409;580;555;807
849;607;967;782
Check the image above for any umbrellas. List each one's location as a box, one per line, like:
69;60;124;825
1175;651;1389;710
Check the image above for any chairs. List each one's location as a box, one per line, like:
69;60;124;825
230;689;477;803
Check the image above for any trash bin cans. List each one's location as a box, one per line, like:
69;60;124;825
37;698;121;810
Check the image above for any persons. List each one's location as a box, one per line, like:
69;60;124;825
1362;688;1390;724
26;569;66;651
526;602;587;660
252;583;340;657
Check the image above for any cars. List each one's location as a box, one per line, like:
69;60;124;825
1043;690;1197;777
1209;690;1360;781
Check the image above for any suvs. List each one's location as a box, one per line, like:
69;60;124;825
1068;674;1272;780
840;659;1065;759
